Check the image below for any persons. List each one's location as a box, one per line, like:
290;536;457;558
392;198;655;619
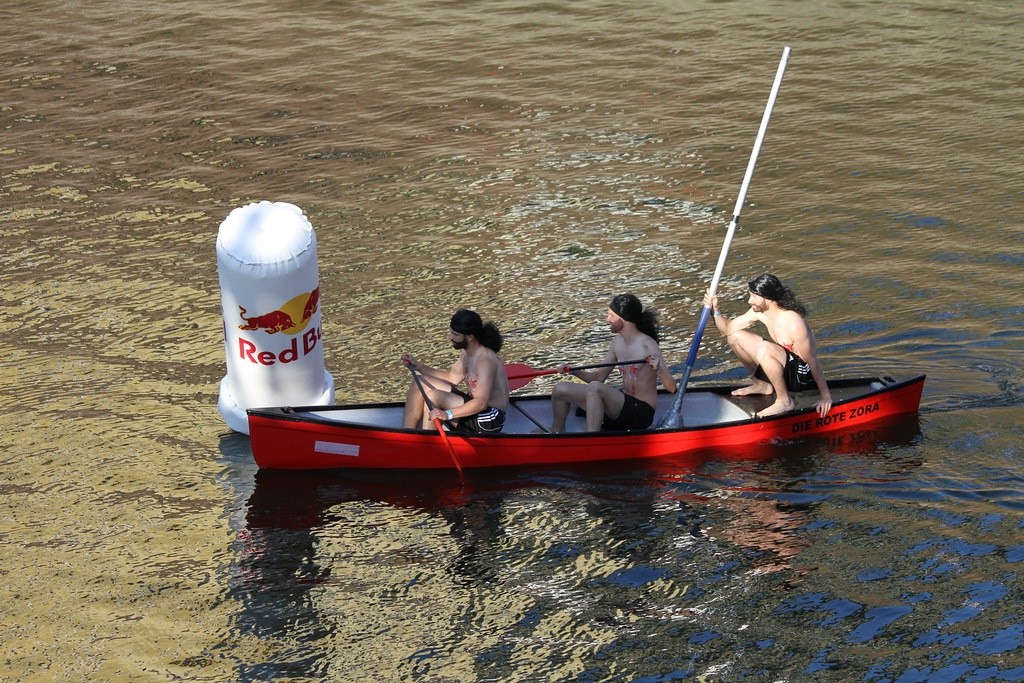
401;310;509;436
530;294;676;435
703;272;832;417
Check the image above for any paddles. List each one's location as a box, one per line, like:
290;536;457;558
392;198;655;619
503;354;653;390
403;356;477;497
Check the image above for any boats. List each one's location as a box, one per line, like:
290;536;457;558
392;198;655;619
246;373;927;471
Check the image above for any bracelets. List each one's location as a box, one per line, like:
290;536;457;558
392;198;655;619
713;311;721;317
572;371;577;375
445;410;452;420
